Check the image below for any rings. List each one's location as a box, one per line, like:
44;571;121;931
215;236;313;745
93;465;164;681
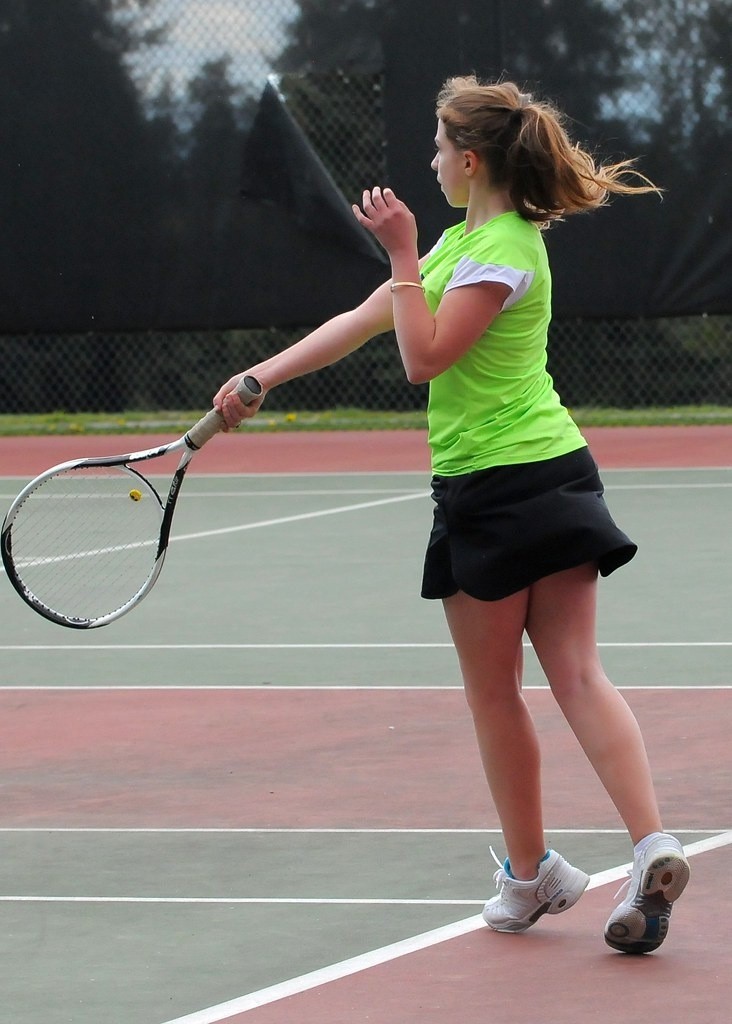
236;423;241;428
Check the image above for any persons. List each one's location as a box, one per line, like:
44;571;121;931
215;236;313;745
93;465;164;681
214;72;692;952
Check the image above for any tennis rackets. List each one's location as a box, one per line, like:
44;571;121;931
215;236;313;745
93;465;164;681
1;373;263;631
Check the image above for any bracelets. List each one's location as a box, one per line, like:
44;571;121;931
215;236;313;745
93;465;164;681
390;282;425;293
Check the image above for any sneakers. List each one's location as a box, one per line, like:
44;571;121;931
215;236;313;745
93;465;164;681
604;834;691;953
481;845;589;933
